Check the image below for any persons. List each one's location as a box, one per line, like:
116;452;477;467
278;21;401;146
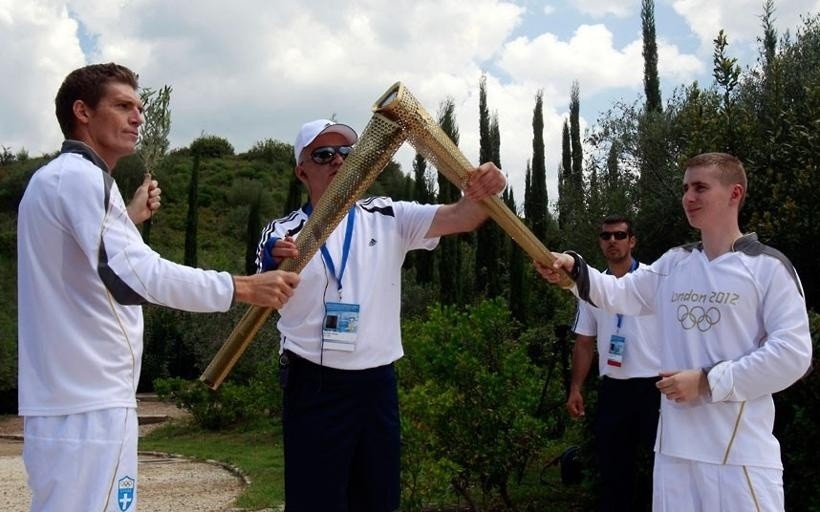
561;211;663;511
17;63;302;511
254;119;509;511
530;151;815;510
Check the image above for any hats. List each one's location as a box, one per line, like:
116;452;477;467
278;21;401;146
293;118;358;166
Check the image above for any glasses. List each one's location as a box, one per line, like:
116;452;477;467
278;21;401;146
599;231;627;240
311;145;355;164
